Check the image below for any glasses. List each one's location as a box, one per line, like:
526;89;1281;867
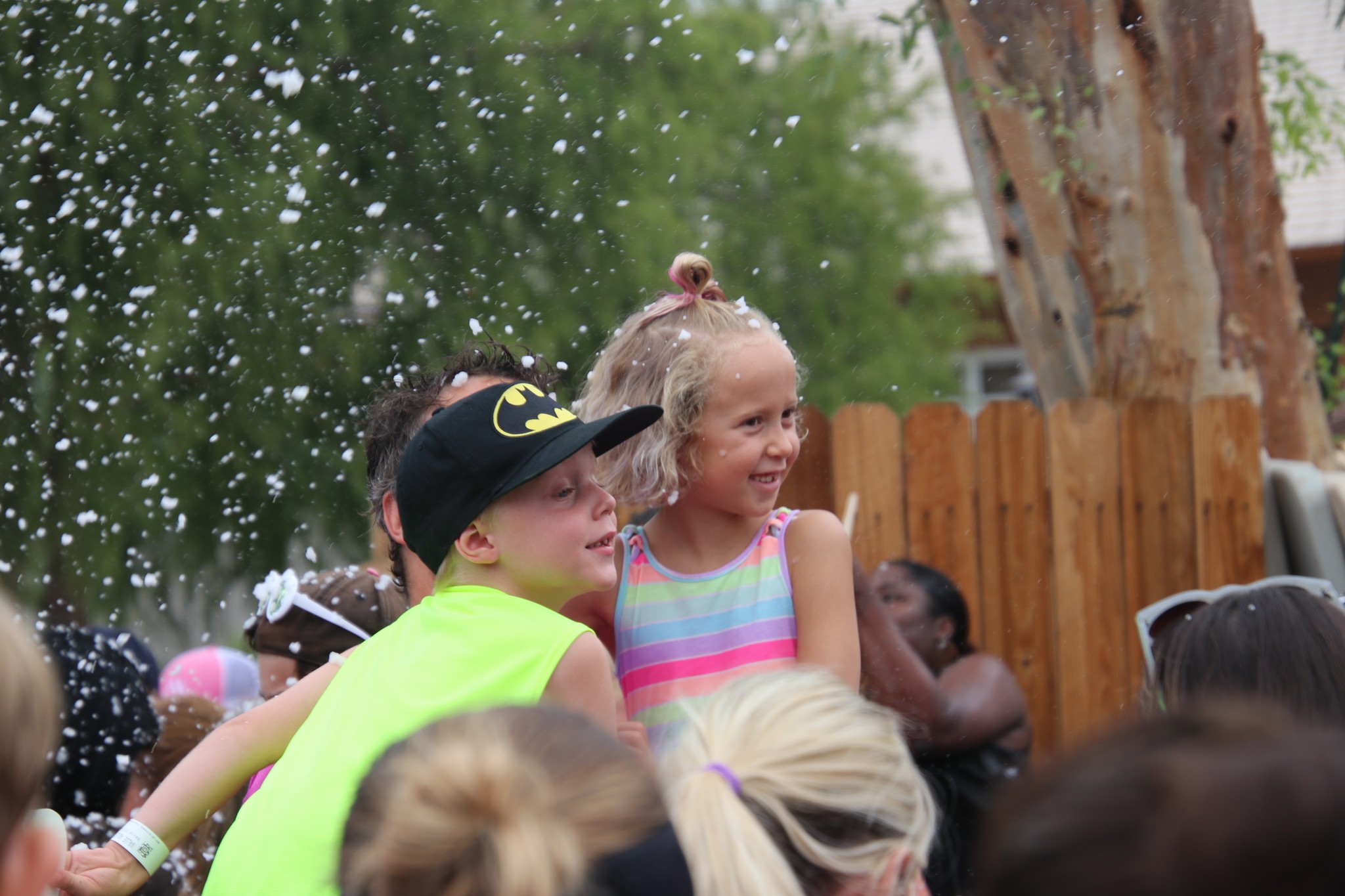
1135;576;1345;713
257;568;372;642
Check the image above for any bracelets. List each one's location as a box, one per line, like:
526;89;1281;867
111;819;170;876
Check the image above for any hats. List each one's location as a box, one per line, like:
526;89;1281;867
399;378;663;574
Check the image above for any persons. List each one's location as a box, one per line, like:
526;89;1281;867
847;558;1029;799
575;251;862;784
0;569;1345;896
50;383;665;896
363;347;616;652
